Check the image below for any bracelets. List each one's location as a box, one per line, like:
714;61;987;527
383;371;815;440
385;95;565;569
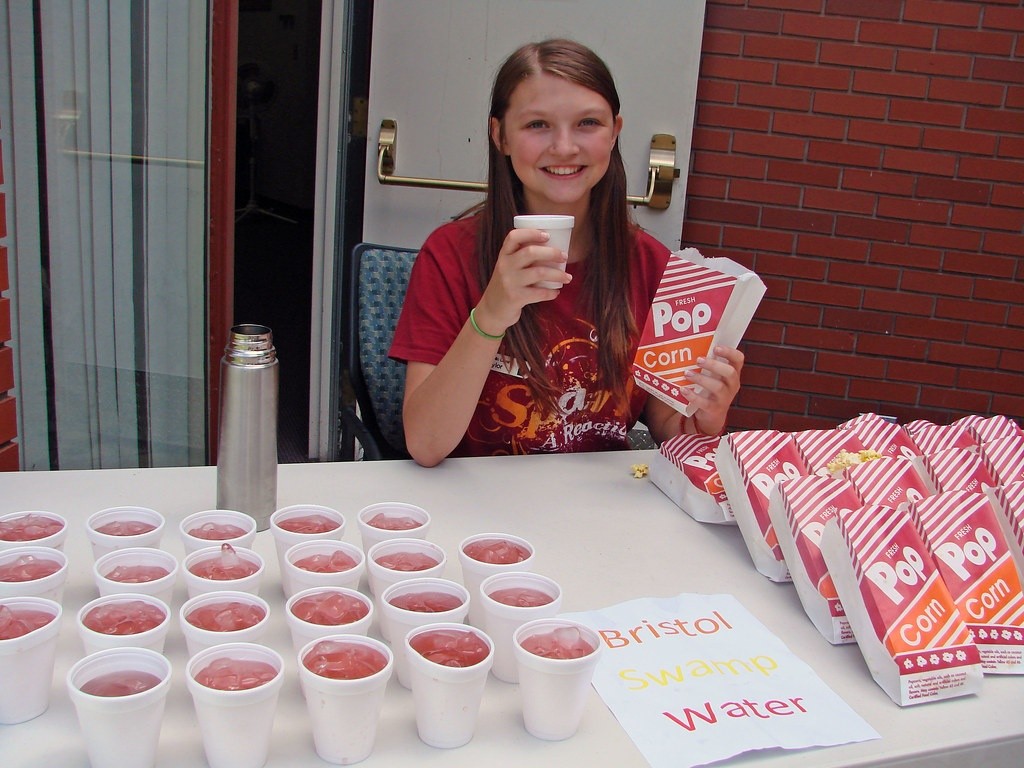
470;308;506;339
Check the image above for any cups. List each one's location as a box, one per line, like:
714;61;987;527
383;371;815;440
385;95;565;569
0;501;604;768
514;213;575;290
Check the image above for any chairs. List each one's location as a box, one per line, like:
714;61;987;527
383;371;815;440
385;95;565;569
340;244;472;461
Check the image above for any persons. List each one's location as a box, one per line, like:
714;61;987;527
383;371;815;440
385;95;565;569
389;38;743;466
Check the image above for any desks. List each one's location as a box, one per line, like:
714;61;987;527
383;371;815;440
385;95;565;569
0;447;1024;768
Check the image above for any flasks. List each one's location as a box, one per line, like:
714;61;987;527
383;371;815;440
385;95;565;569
217;322;279;533
